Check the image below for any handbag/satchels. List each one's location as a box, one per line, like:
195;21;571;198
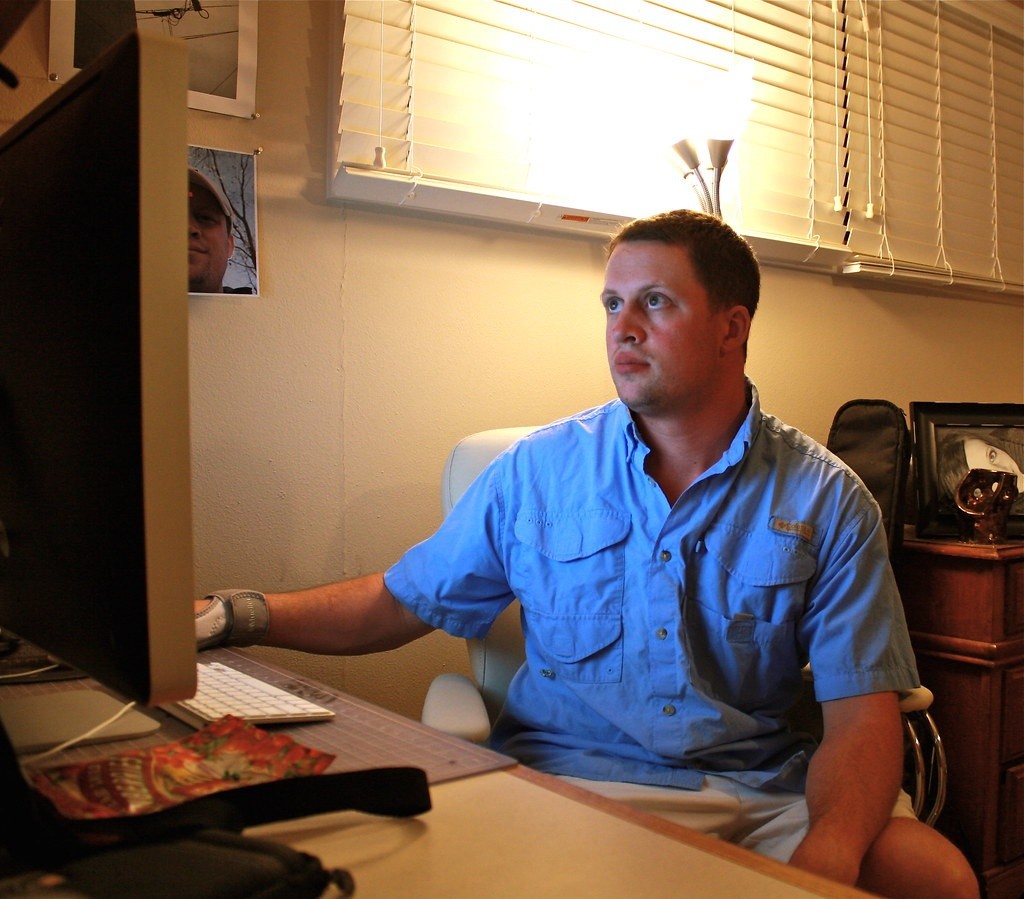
59;828;355;899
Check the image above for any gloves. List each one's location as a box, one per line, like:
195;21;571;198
196;588;270;649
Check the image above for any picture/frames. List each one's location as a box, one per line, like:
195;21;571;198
907;400;1024;538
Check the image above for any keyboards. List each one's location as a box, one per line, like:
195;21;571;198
153;663;335;732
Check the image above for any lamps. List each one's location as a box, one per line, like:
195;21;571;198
674;136;733;222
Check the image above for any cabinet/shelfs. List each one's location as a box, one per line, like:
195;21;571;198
896;534;1024;899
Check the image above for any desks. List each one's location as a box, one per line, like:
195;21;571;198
0;650;882;899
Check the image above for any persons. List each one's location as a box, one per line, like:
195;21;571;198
195;209;982;899
186;168;251;295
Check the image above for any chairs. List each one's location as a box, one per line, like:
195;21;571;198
418;429;946;830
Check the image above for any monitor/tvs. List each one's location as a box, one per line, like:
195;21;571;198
0;25;197;754
